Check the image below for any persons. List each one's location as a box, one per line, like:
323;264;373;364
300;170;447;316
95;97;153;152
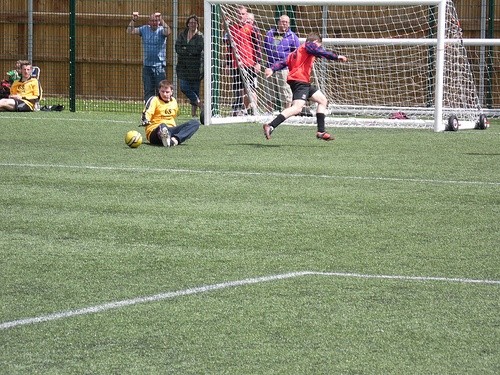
0;61;42;112
125;12;171;105
263;31;347;141
263;15;301;113
5;59;32;91
221;5;262;117
175;14;204;117
139;80;199;147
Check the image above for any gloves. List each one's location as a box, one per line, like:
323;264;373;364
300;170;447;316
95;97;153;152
138;119;152;127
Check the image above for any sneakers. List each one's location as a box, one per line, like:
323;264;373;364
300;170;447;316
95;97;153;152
263;124;272;141
157;123;177;148
317;130;334;141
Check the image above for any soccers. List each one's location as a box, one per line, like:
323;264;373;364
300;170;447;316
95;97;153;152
125;131;142;148
